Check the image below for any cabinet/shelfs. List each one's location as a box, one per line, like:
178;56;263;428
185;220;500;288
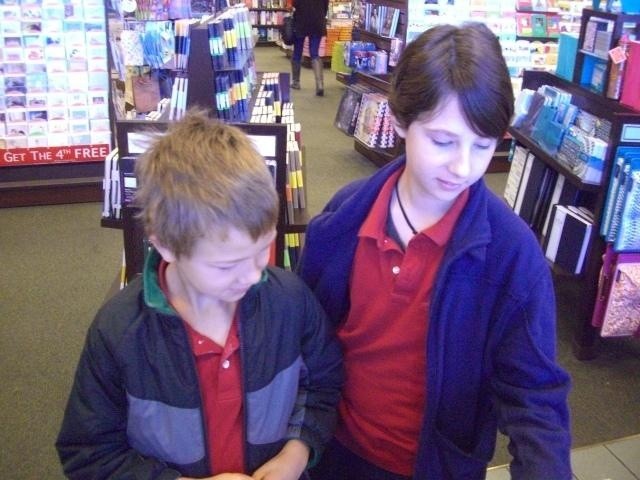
100;0;362;286
348;0;639;359
1;1;112;209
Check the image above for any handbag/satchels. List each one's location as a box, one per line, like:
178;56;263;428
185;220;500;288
280;16;295;45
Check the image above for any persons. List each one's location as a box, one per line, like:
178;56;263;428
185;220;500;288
300;25;573;480
55;114;346;480
288;0;329;96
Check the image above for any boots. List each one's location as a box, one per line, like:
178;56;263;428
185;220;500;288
290;60;301;89
310;55;324;96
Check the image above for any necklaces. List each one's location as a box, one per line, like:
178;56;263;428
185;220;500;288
394;181;421;237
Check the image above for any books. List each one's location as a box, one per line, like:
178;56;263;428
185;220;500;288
500;145;595;276
